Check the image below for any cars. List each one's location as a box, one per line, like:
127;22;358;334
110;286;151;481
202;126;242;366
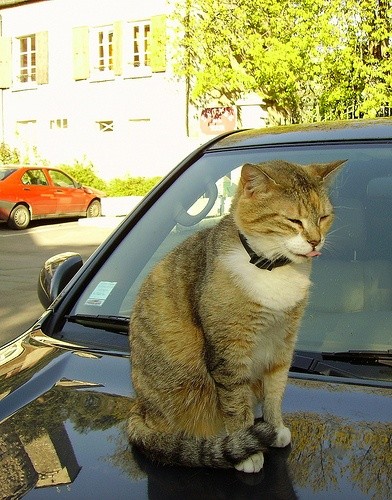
0;116;392;500
0;164;102;230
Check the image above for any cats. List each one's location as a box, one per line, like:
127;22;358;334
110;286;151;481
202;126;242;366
124;158;348;472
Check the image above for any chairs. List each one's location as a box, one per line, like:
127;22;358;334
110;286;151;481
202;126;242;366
307;177;392;312
